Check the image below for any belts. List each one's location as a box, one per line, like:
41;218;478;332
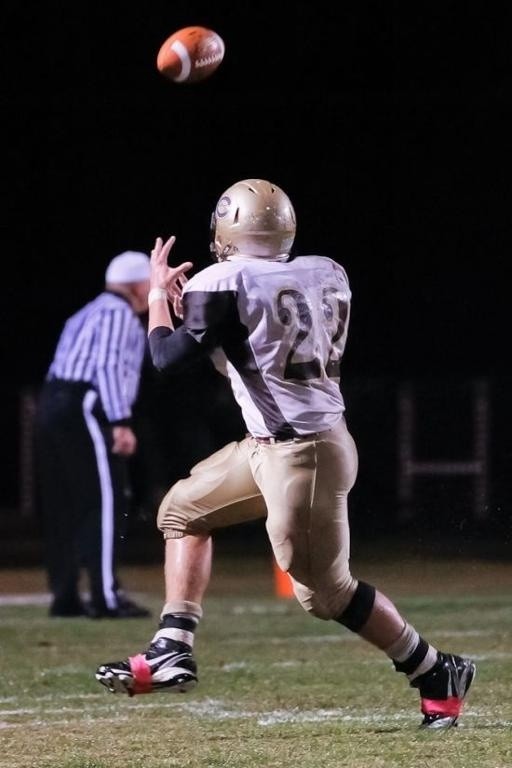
256;432;319;444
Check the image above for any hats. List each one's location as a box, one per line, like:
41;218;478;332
106;251;151;283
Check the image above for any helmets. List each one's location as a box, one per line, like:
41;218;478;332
210;179;297;261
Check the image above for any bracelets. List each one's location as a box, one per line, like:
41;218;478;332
146;288;166;304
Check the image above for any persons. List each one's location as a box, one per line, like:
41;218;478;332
39;244;150;620
94;176;475;729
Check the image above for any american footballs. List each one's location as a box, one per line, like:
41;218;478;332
158;26;225;84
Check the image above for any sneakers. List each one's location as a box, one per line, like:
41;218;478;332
89;598;151;618
410;651;476;731
47;598;90;617
95;637;198;697
